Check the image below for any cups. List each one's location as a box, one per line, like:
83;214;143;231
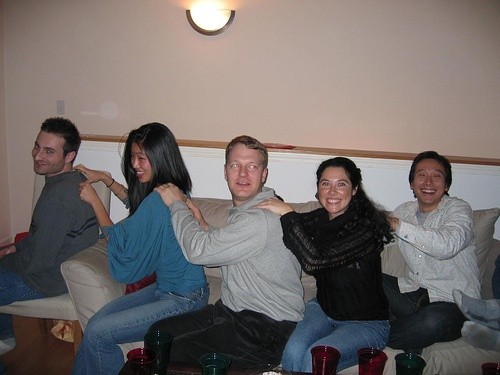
357;347;388;375
127;347;158;375
143;330;175;375
394;353;426;375
198;353;231;375
310;346;341;375
481;362;500;375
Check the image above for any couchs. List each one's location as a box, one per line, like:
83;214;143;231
62;198;500;375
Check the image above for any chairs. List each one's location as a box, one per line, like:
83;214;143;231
0;173;112;358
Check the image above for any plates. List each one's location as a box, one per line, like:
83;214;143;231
244;364;293;375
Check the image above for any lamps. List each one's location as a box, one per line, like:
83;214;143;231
185;10;236;35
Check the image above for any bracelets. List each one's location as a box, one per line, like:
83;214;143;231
115;185;125;195
107;179;114;188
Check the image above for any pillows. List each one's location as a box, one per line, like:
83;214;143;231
382;207;500;284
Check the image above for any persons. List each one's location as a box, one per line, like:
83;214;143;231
142;136;305;375
380;151;482;350
72;122;211;375
0;119;99;355
452;253;500;352
255;157;395;373
